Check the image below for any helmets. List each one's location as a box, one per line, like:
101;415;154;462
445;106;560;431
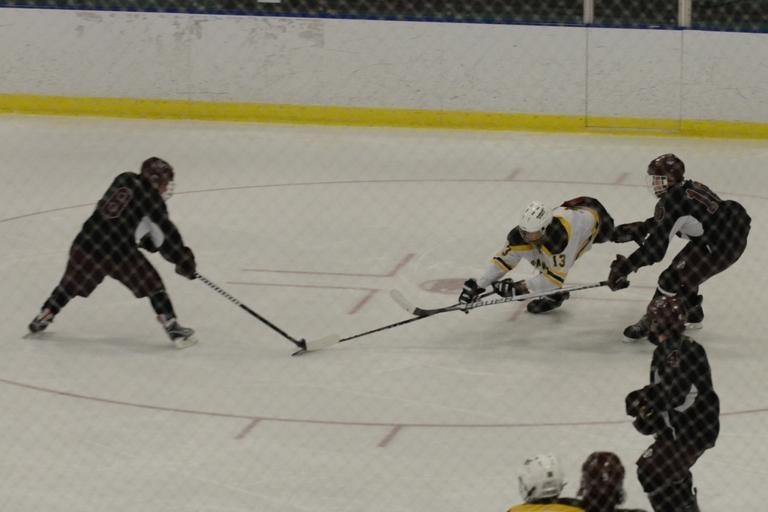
580;450;626;507
141;156;175;201
643;153;685;198
520;455;569;504
519;199;552;234
645;295;685;344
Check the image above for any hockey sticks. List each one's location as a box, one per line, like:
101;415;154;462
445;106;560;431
389;280;628;317
192;271;339;351
291;279;529;357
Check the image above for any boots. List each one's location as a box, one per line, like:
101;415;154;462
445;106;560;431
157;312;194;341
687;295;704;325
28;304;58;332
624;313;652;339
526;291;569;314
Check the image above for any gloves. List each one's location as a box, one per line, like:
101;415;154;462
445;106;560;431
175;248;196;280
140;231;159;254
491;278;527;297
607;255;632;292
459;279;484;313
626;384;666;415
633;414;661;434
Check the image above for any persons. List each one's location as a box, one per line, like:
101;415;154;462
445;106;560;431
571;452;644;511
611;153;749;339
458;196;612;316
505;453;595;511
623;296;721;512
28;156;195;345
